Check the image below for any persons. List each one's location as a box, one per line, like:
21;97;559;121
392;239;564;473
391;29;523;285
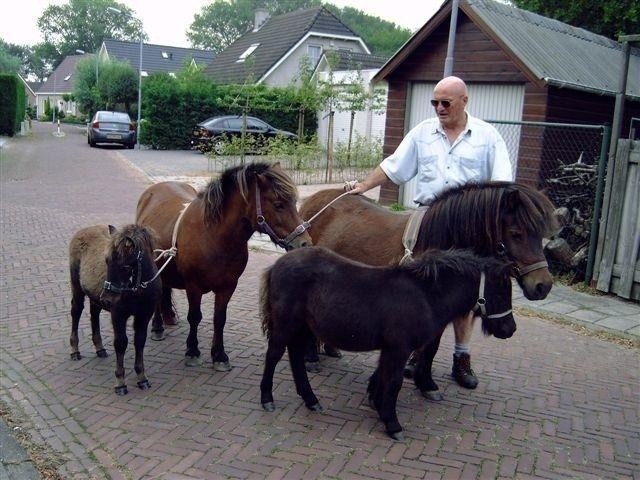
344;76;512;390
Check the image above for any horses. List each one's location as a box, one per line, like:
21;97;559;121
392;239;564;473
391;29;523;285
294;178;556;401
258;247;518;443
134;158;314;372
67;222;164;397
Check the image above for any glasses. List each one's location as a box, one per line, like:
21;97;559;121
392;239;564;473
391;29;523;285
430;97;457;108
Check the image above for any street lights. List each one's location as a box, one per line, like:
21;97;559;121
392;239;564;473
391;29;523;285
106;7;142;150
74;49;99;85
47;71;56;123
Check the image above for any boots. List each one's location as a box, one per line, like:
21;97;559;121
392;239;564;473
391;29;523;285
404;350;417;379
452;353;480;388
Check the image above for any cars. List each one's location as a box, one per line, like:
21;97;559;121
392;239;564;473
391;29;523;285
87;111;136;149
190;115;298;154
